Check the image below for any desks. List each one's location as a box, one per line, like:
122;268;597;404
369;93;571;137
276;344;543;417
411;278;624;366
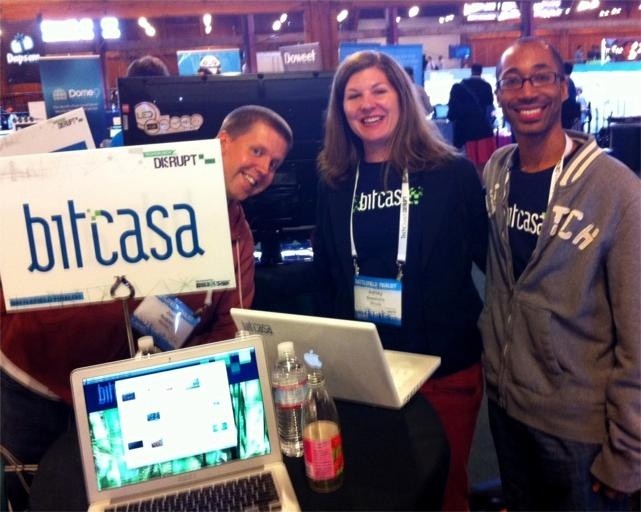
22;387;455;510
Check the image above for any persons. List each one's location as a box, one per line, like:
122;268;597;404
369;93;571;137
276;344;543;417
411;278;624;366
481;35;641;511
561;61;581;130
446;63;496;149
574;38;641;63
97;56;170;147
422;52;445;70
1;104;294;511
311;50;490;511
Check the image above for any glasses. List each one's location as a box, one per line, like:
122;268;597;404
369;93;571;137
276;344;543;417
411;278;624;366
496;71;565;90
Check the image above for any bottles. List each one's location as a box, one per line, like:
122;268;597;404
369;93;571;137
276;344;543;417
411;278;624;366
269;340;313;460
133;335;162;360
299;369;347;493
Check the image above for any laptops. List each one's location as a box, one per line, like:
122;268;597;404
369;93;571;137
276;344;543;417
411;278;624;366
229;307;443;409
68;334;304;512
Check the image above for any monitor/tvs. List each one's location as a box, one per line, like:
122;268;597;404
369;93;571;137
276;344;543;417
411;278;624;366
117;74;346;243
